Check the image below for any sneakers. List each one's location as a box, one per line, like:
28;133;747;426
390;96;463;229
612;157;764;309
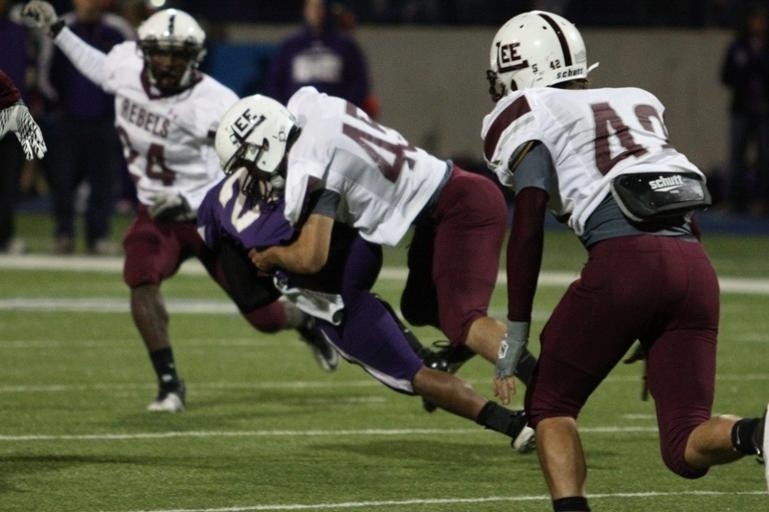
298;313;341;374
423;350;462;415
750;404;769;488
147;381;186;414
427;336;477;363
507;410;536;453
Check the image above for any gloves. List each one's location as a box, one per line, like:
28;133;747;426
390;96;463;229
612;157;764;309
147;194;186;222
14;1;61;39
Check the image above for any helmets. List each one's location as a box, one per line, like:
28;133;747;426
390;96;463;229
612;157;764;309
134;8;207;93
485;9;599;103
214;94;297;214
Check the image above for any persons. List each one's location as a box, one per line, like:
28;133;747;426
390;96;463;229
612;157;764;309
215;86;535;413
265;0;366;107
480;10;767;512
24;0;339;414
720;12;765;213
197;136;542;453
2;1;145;257
